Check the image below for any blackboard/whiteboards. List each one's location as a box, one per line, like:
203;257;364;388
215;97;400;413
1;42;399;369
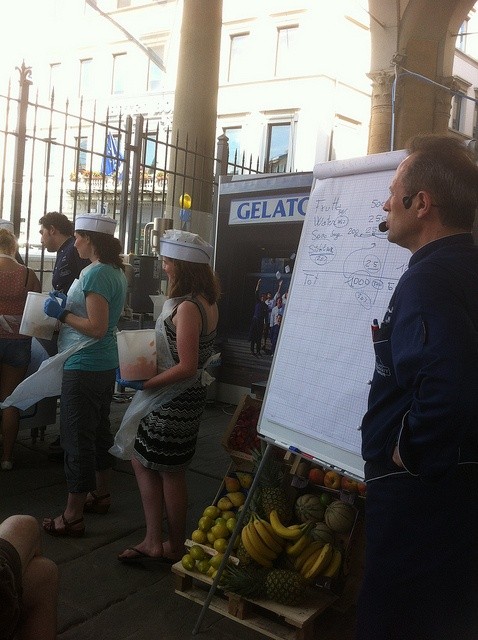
255;136;478;480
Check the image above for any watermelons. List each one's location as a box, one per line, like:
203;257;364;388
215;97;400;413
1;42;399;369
293;494;357;545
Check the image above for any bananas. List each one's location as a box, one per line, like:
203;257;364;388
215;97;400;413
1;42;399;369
269;509;314;556
294;541;342;578
241;511;284;567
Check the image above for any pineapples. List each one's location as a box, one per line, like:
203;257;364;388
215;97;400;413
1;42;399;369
260;464;294;523
235;542;259;568
218;568;306;605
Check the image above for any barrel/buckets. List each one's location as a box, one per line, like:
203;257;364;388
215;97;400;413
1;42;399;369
18;291;63;341
115;328;159;382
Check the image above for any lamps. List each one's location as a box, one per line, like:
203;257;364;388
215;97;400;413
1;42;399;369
392;53;407;65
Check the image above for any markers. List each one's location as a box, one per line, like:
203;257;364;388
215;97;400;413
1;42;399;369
274;440;299;453
304;453;347;472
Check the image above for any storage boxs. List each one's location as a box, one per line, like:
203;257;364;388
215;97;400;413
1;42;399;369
184;394;368;595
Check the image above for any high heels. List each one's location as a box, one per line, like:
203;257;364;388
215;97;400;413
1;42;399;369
44;514;86;537
83;490;111;514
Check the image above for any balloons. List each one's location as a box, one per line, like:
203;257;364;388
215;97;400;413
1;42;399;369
178;194;191;221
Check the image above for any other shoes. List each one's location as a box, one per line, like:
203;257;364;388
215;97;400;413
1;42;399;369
2;461;13;471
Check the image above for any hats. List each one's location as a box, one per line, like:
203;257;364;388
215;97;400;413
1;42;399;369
0;219;14;234
159;230;214;264
75;213;117;235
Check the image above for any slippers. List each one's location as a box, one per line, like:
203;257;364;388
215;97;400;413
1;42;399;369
163;559;178;564
117;547;162;561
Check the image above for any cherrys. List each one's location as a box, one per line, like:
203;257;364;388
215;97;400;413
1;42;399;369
229;406;264;454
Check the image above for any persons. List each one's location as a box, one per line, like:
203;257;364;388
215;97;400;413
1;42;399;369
44;214;128;537
0;228;41;469
40;212;92;296
0;515;57;640
354;133;478;640
120;228;218;561
250;278;287;357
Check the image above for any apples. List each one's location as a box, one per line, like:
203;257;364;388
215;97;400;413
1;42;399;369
307;468;366;494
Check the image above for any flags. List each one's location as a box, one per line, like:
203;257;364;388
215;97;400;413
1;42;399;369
100;132;124;176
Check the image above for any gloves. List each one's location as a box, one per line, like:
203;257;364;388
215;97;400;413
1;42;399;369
53;290;66;310
44;292;64;320
116;368;143;390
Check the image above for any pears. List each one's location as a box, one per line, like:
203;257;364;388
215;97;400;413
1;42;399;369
181;471;252;580
